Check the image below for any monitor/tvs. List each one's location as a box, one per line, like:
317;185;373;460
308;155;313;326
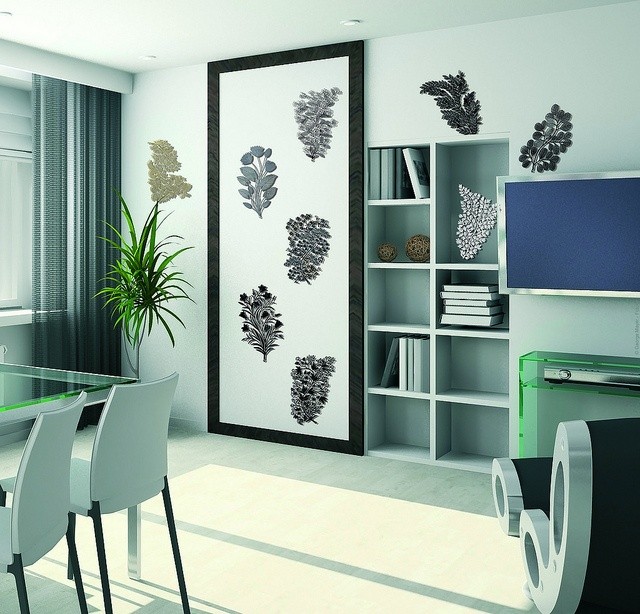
496;171;640;299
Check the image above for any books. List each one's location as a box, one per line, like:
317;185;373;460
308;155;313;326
368;148;429;199
381;335;429;394
439;284;505;328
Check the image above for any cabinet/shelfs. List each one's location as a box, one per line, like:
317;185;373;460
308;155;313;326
518;350;640;459
365;133;511;476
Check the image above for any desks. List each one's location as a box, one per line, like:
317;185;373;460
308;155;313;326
1;361;142;582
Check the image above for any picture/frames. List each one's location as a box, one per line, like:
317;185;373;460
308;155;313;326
207;39;365;457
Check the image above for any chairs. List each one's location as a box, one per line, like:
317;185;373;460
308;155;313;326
0;391;89;614
0;371;191;614
490;418;639;614
